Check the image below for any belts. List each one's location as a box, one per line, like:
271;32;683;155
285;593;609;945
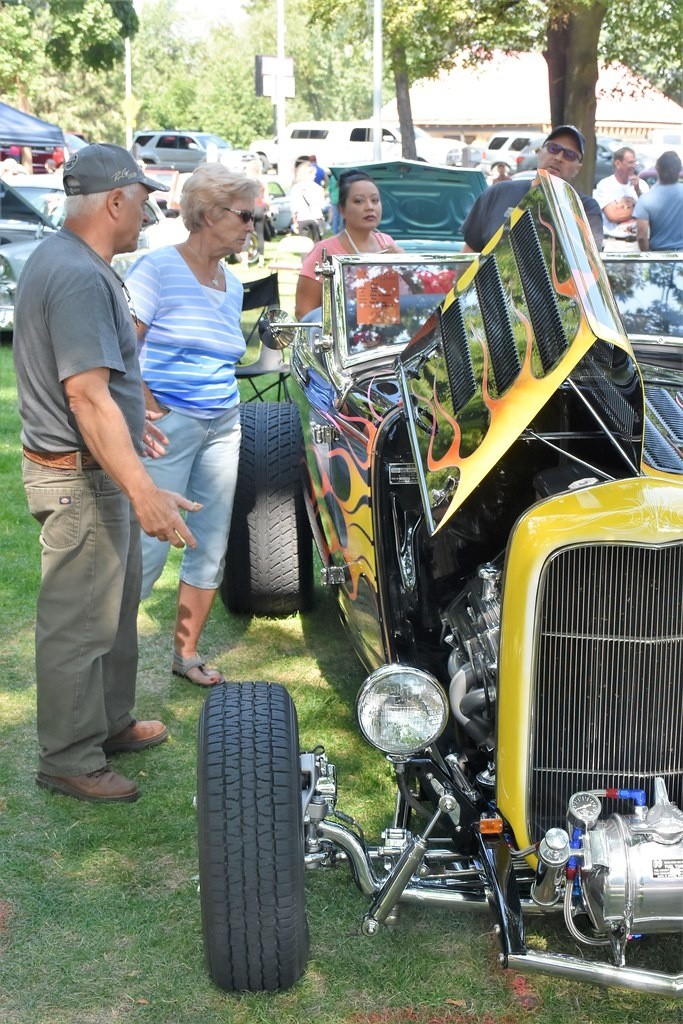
22;445;103;470
608;235;638;242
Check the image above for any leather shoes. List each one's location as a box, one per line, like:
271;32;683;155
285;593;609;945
101;721;167;756
36;766;140;805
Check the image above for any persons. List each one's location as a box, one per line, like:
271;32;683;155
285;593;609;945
294;169;404;324
458;124;603;254
594;147;649;252
11;145;204;802
292;155;345;266
633;151;683;251
119;163;260;688
494;165;512;185
244;160;264;258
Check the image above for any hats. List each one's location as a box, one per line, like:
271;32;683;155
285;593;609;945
543;123;586;162
63;143;170;196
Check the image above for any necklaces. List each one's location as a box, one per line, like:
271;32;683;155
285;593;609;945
186;243;219;286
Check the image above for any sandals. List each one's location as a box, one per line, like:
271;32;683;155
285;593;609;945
172;653;225;686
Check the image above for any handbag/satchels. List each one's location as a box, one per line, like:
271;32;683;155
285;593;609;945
316;218;326;237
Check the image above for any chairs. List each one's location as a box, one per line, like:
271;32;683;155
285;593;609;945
231;270;294;404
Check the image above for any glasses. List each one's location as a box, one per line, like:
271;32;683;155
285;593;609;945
543;143;581;163
222;207;255;223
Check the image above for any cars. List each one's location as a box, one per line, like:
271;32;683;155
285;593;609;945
195;164;682;1014
0;119;683;338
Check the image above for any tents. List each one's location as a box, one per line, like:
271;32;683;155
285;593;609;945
0;101;69;160
